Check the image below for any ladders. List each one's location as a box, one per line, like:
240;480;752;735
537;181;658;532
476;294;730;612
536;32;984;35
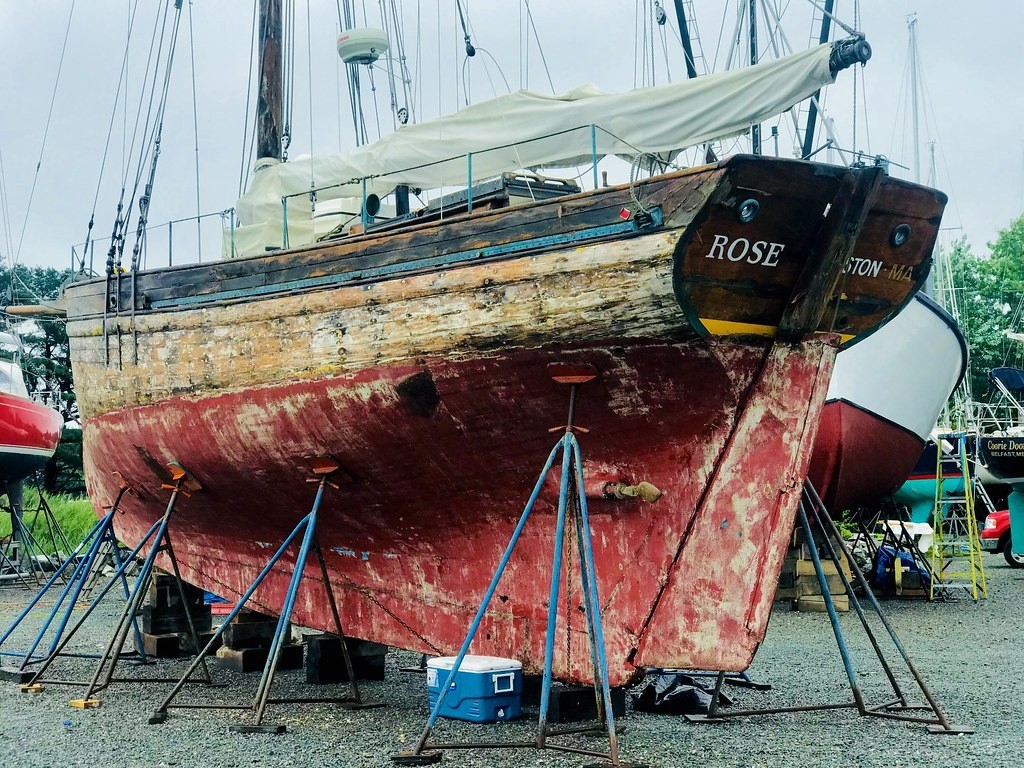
930;433;989;605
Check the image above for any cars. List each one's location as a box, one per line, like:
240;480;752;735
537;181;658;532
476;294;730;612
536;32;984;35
980;510;1023;566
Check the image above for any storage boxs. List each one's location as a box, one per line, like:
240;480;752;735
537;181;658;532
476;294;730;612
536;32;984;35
883;522;934;553
427;655;524;723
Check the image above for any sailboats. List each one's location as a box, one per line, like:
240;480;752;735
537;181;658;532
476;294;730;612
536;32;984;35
57;3;947;679
762;12;966;520
